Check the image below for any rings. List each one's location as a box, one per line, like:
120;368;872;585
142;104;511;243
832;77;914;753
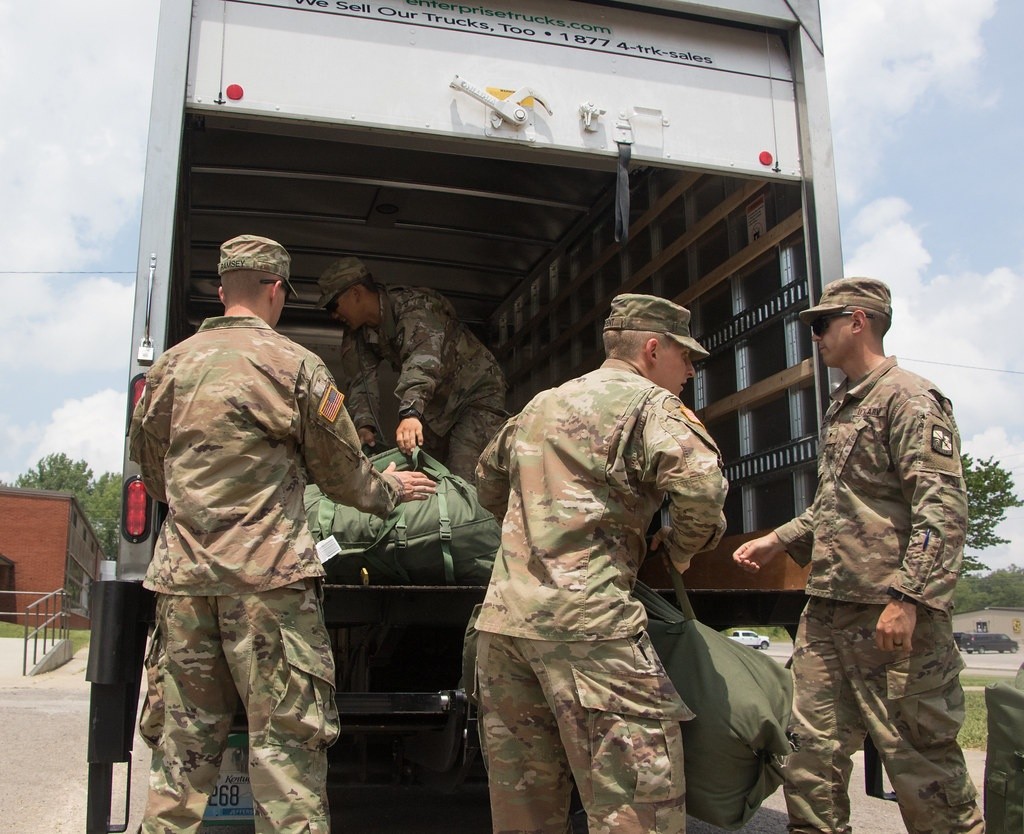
894;641;902;647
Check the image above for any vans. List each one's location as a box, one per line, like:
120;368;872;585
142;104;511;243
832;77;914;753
961;633;1019;653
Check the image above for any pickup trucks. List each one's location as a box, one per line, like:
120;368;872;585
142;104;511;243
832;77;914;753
727;630;770;650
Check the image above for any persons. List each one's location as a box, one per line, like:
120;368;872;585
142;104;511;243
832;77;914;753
475;294;728;834
317;257;514;486
733;275;986;834
129;234;437;833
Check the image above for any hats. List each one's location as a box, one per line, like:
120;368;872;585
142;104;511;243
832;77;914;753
217;235;299;300
799;277;892;327
316;256;369;309
602;293;710;362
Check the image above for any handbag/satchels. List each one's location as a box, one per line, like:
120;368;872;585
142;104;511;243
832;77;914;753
462;535;801;831
983;661;1024;834
304;445;501;584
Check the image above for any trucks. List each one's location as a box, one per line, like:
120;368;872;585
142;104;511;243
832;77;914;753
85;2;843;832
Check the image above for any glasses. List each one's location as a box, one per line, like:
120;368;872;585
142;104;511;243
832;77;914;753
259;280;292;303
810;311;873;336
327;278;369;313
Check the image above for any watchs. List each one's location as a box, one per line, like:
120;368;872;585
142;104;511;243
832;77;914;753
887;588;918;605
